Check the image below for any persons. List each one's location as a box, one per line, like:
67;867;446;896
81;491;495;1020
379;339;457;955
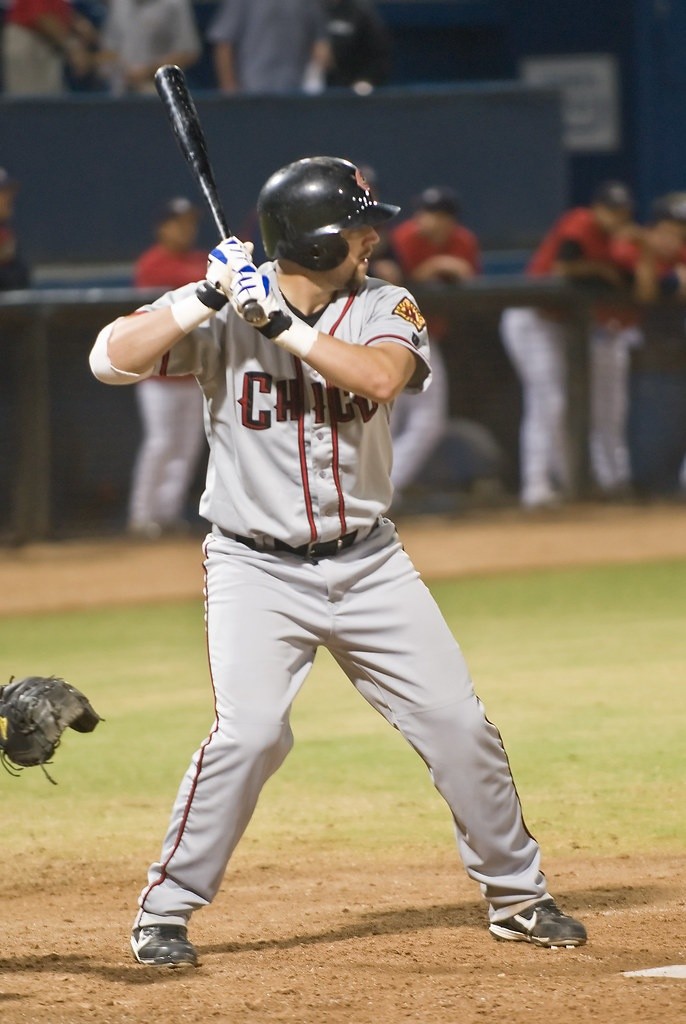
126;195;217;541
496;183;686;511
88;154;586;969
373;187;486;510
0;0;339;92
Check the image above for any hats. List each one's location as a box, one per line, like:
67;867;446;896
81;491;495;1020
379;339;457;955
591;180;636;212
162;197;200;215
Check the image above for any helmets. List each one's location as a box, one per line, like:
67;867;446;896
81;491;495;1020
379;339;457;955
257;155;402;272
417;186;461;214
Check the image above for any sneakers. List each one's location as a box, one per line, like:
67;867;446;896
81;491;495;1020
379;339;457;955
129;924;197;968
489;899;588;946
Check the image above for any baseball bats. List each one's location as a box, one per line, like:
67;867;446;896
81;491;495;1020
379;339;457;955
154;62;264;325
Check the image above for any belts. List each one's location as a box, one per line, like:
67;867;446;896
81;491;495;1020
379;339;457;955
275;530;357;559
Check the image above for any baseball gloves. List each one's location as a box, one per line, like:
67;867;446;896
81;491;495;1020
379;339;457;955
0;674;106;785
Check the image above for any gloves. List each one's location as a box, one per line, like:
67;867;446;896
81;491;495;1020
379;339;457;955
226;271;294;340
196;235;257;312
